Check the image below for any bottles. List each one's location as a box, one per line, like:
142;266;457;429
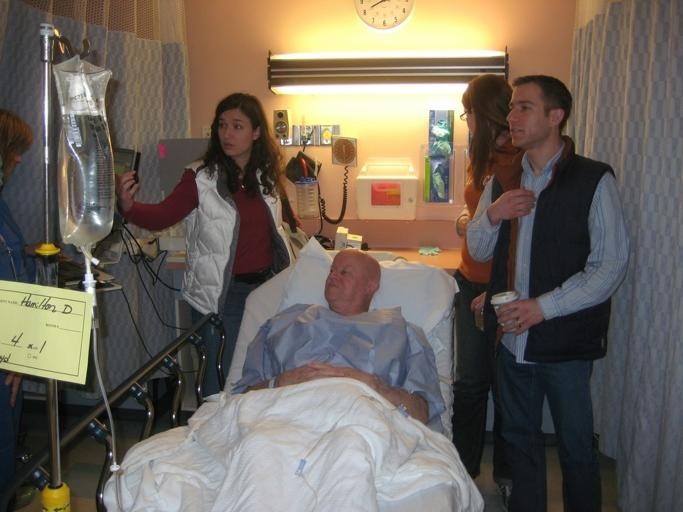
294;176;317;219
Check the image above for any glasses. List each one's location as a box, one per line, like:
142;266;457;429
460;107;472;120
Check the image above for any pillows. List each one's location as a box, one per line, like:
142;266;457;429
278;237;459;334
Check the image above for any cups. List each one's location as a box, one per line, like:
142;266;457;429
490;291;520;333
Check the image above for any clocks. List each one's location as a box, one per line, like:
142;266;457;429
355;0;415;29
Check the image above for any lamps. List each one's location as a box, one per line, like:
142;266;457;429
267;46;509;95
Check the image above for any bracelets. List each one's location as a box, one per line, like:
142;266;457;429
267;376;277;388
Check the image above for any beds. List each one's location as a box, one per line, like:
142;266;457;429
0;250;485;511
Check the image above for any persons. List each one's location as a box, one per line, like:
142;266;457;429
454;76;522;505
234;248;447;424
467;77;628;512
116;92;307;394
0;109;37;512
